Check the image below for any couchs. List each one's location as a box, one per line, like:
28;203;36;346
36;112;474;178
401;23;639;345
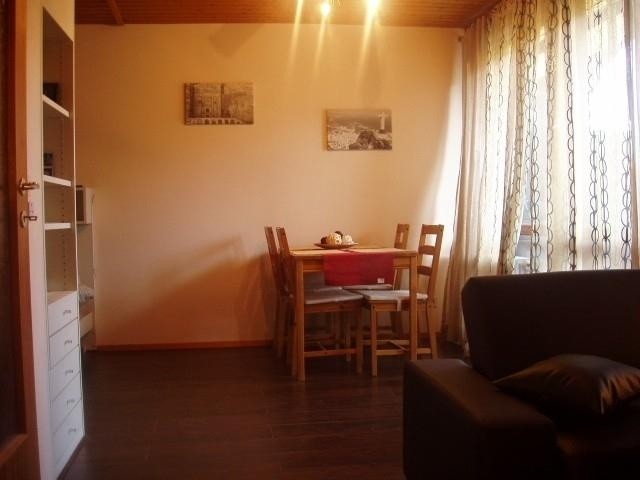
400;268;640;480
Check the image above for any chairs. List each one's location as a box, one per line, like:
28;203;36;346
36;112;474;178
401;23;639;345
275;226;365;382
356;223;445;377
263;226;343;358
345;223;410;361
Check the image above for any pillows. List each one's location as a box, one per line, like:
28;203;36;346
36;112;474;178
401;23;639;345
488;348;640;437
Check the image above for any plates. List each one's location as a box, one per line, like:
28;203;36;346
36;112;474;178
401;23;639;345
313;242;360;250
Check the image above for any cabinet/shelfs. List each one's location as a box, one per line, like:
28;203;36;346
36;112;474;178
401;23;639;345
35;4;78;303
43;291;87;480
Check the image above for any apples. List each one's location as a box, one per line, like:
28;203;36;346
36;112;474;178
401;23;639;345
321;231;352;244
